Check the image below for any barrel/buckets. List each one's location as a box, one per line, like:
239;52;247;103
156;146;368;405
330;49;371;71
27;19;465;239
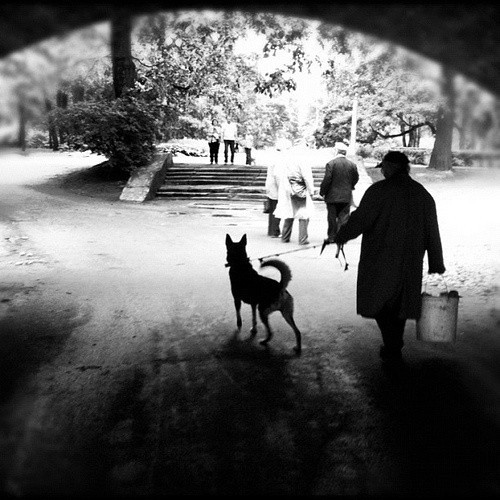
416;272;458;343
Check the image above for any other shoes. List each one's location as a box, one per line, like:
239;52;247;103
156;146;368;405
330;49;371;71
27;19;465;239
271;235;282;237
304;239;310;244
380;347;404;360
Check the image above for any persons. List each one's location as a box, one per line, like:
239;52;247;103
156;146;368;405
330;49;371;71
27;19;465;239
264;139;294;237
221;115;238;166
242;128;254;166
207;117;222;166
320;148;360;243
330;150;447;362
272;137;316;246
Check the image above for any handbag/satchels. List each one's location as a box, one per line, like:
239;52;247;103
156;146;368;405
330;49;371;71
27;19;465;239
289;175;308;200
264;197;278;213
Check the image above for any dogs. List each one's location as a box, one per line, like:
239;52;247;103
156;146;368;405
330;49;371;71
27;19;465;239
225;233;302;355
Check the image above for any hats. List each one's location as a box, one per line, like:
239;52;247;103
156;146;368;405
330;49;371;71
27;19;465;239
334;142;348;152
375;151;410;168
